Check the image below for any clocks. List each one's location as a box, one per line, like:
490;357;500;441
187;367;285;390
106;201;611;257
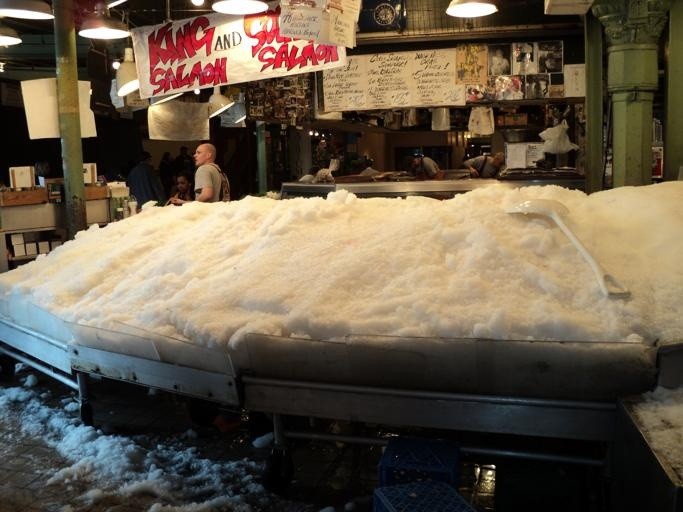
374;3;396;26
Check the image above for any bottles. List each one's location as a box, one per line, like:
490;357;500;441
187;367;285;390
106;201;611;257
116;195;138;220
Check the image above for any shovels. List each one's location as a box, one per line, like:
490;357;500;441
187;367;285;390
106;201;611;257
507;199;630;298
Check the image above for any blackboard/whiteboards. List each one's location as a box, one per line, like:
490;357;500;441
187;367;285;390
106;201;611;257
321;46;466;111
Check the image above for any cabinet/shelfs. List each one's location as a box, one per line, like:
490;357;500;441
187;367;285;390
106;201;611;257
0;199;110;272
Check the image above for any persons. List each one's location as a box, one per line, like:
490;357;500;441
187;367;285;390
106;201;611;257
462;152;505;178
410;153;444;180
128;151;166;208
174;146;195;179
169;175;195;206
159;151;178;200
193;143;223;202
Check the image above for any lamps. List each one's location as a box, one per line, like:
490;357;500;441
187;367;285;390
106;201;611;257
208;87;234;118
0;0;56;22
212;0;269;16
0;26;22;47
445;0;499;18
106;0;127;8
115;38;140;96
227;102;247;123
77;4;130;39
